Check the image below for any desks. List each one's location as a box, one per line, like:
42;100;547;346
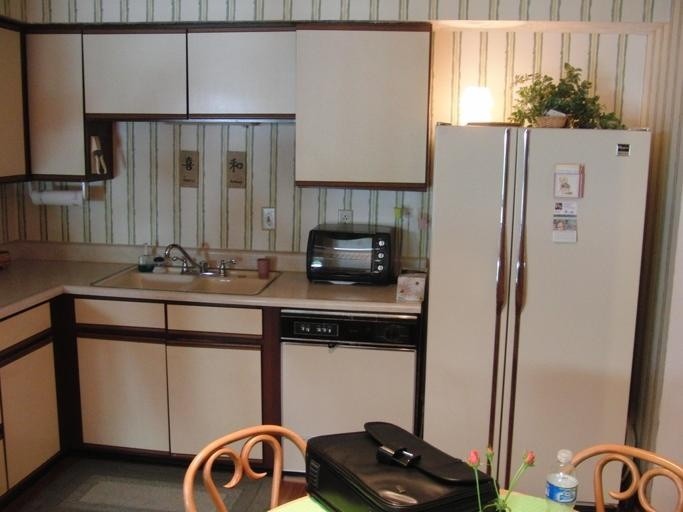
267;488;577;512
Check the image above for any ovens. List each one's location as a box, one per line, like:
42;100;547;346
305;224;397;286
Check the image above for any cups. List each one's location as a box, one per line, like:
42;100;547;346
258;258;270;279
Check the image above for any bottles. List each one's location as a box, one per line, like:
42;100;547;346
138;243;166;273
545;449;578;512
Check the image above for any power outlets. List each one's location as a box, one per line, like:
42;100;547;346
262;207;276;231
339;210;353;224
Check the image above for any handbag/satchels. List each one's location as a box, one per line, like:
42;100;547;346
303;418;498;511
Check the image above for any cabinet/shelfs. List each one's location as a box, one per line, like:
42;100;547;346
0;296;263;512
296;29;432;184
85;31;295;116
2;27;25;178
26;32;86;179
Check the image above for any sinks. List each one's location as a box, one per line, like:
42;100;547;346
190;275;271;295
91;271;194;291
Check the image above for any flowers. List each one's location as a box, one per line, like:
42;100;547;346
467;446;536;512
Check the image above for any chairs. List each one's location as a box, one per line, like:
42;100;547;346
565;445;683;512
182;425;307;512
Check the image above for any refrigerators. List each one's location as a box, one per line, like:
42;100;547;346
424;123;651;508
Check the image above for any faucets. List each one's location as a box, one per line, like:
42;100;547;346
165;243;200;268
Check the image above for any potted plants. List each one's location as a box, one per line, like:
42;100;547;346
508;63;627;130
